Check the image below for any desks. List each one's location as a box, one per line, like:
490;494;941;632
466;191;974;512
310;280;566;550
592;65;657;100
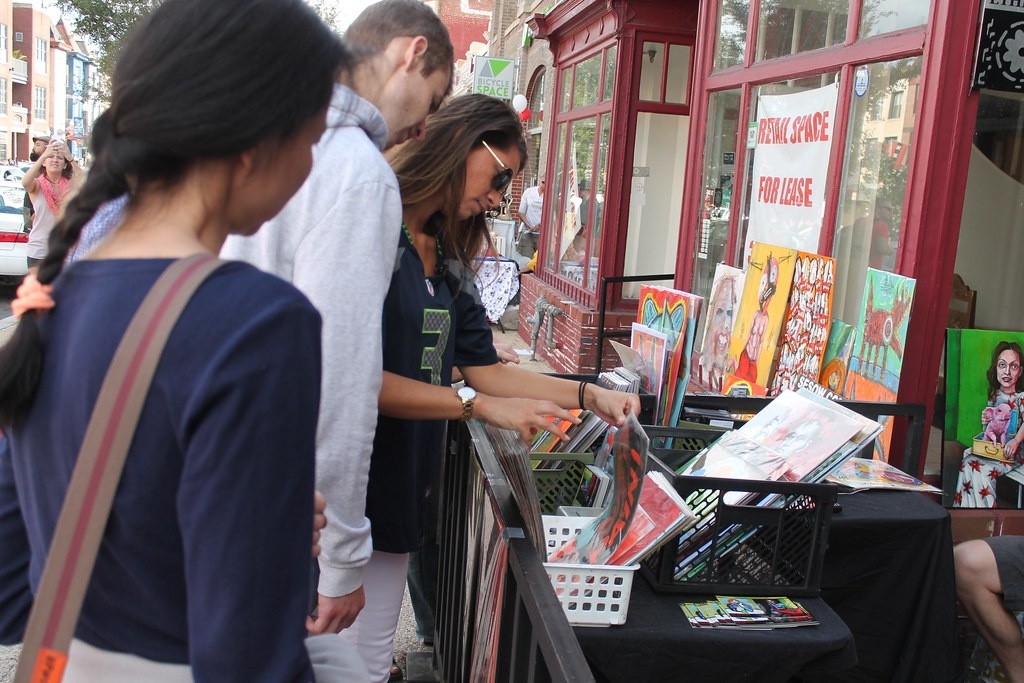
582;464;957;683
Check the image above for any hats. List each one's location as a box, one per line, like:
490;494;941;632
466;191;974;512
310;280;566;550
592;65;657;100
32;134;50;142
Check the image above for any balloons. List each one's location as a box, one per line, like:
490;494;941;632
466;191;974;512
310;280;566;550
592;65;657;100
538;109;544;122
519;107;530;121
512;94;530;113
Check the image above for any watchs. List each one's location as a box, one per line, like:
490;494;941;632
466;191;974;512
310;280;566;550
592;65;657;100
456;387;481;422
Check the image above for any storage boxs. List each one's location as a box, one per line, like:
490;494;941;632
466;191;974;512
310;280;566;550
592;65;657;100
506;361;838;600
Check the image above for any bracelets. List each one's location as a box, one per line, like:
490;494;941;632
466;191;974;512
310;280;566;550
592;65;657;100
578;380;589;411
69;158;75;163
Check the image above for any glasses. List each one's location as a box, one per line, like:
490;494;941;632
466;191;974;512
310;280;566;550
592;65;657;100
479;137;514;198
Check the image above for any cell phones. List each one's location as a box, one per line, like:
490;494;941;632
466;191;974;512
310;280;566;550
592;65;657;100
51;133;65;145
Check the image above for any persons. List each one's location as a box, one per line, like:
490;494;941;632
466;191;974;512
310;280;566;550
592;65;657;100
573;180;600;261
21;137;84;272
953;533;1024;682
302;89;647;682
517;176;546;260
0;0;351;683
214;0;458;643
22;135;51;240
832;197;895;326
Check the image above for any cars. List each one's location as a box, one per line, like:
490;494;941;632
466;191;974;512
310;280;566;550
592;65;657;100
16;161;35;172
710;206;747;223
1;164;30;191
1;184;29;289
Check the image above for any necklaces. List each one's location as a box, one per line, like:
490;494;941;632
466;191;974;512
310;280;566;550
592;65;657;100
44;175;63;185
